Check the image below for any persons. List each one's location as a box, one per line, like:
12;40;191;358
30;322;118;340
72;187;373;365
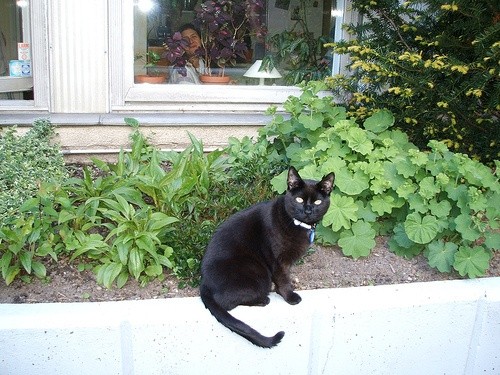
169;23;208;84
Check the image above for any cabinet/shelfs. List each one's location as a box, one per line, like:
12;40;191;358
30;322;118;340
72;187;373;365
147;39;171;82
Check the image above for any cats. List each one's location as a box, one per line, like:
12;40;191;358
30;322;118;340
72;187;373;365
200;166;335;349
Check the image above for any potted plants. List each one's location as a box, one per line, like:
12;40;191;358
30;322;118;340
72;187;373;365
161;0;268;85
133;50;167;83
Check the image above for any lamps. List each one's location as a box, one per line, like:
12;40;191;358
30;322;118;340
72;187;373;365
243;59;282;85
